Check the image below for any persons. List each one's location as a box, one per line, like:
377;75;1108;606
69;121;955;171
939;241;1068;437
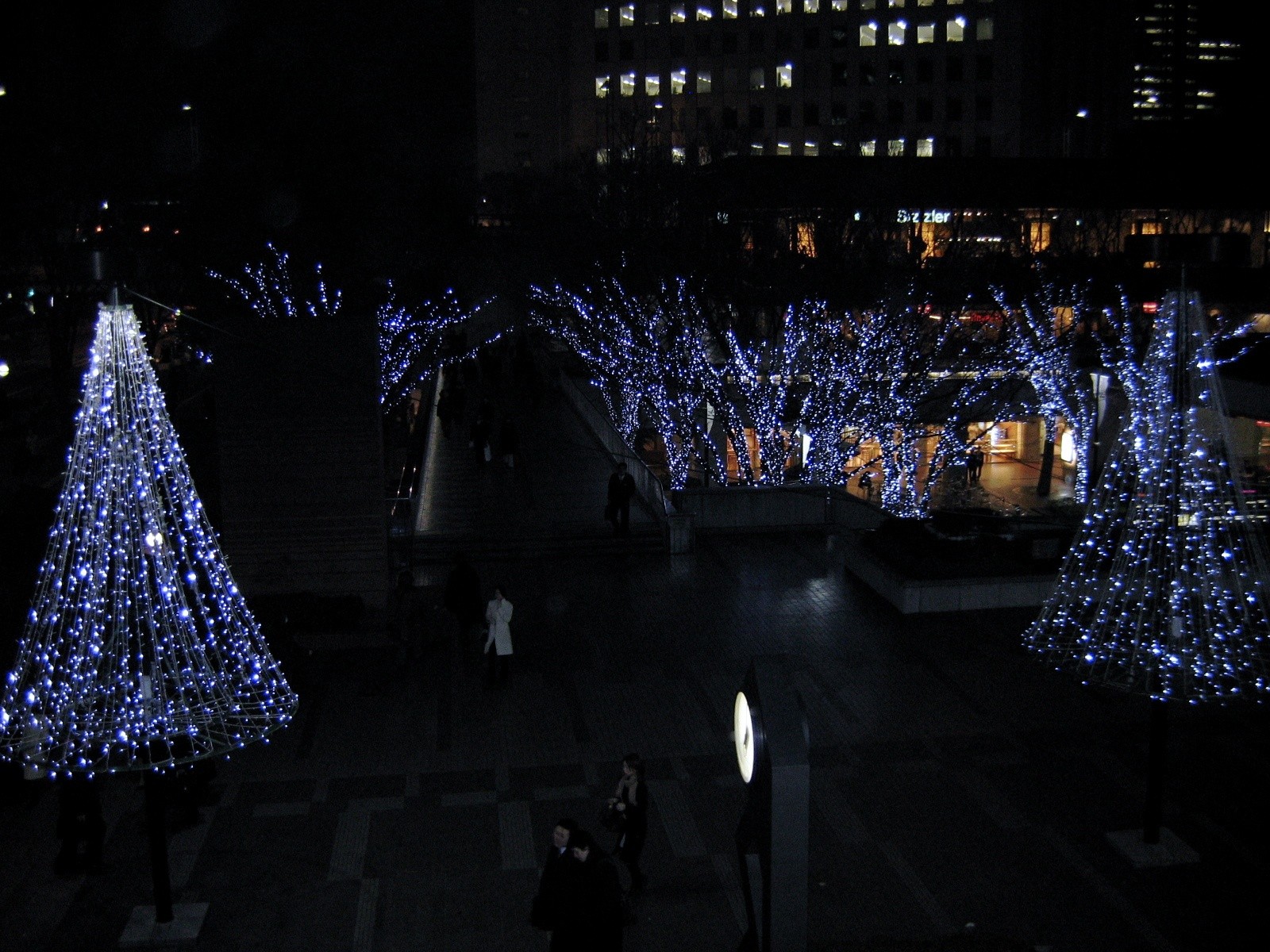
480;586;514;685
967;446;984;483
530;817;622;951
601;753;649;899
53;765;109;878
858;471;872;502
607;462;636;539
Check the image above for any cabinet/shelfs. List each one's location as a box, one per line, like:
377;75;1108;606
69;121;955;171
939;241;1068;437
966;439;1018;463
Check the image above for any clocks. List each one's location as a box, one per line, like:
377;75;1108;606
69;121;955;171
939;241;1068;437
734;690;764;783
